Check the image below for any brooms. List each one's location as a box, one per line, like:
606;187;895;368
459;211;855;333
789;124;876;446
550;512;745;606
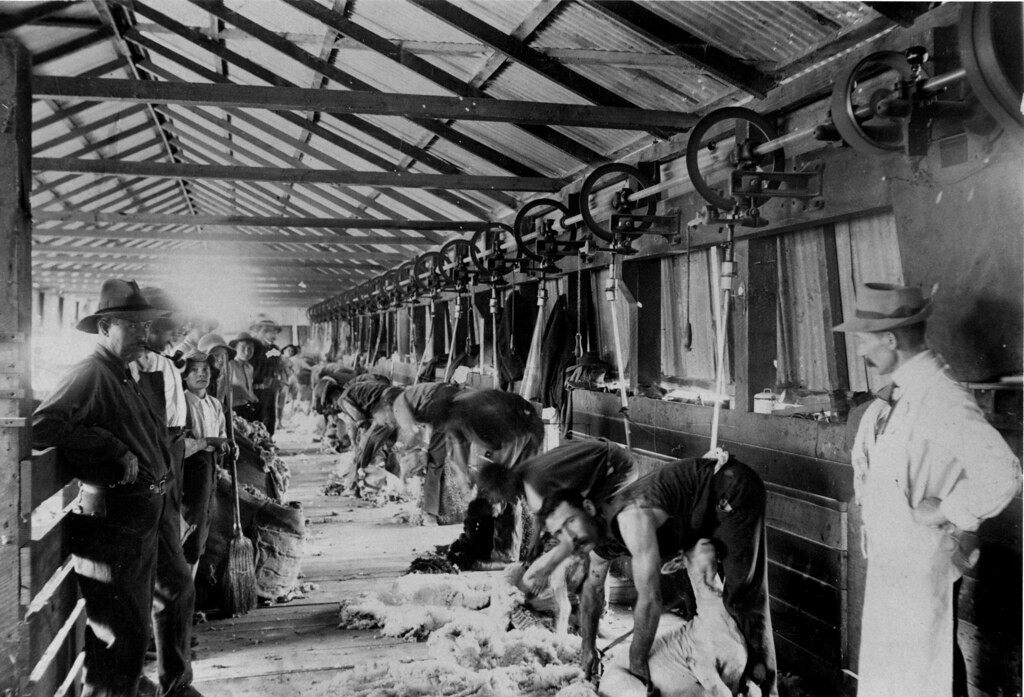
219;352;258;619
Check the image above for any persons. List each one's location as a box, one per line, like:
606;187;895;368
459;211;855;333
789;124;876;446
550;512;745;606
32;275;779;697
831;282;1024;697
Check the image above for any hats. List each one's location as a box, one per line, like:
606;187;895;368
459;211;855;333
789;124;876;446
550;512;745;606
248;320;282;334
280;344;297;357
230;332;263;355
74;279;172;333
198;333;237;360
137;287;185;319
176;349;220;381
832;283;934;333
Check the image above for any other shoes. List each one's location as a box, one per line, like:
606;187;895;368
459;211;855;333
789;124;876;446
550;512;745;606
135;673;157;697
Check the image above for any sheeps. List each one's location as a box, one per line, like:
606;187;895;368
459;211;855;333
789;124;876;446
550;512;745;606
597;538;747;697
